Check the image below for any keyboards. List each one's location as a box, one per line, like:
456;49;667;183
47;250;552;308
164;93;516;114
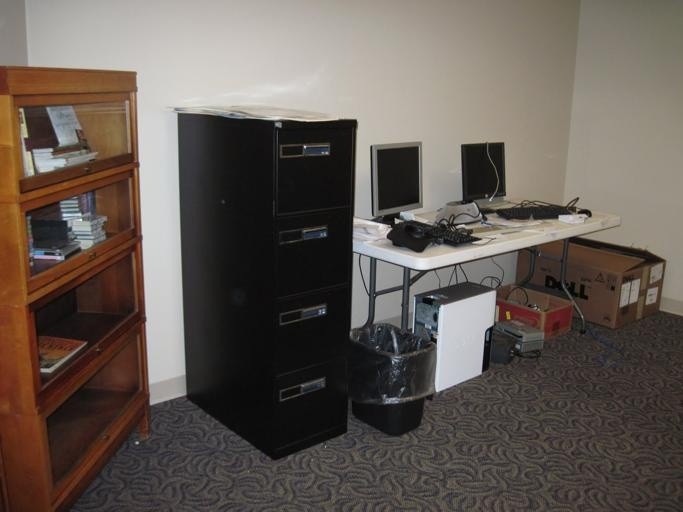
403;219;481;245
498;206;576;220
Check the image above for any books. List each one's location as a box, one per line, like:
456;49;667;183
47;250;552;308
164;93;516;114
26;215;35;267
33;248;81;261
20;106;100;177
37;335;88;373
60;192;108;250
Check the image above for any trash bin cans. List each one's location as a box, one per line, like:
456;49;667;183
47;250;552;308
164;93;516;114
350;324;435;436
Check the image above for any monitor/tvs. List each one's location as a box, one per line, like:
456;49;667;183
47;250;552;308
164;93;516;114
370;142;423;225
462;143;506;213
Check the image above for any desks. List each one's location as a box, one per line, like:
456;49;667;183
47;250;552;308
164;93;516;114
352;198;620;333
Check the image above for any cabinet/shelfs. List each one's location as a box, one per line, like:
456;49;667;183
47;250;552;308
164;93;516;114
177;113;356;461
1;66;152;511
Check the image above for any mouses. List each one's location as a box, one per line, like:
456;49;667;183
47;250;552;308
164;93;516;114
578;210;591;218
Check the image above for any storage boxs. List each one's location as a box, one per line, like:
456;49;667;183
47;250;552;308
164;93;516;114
494;283;574;339
517;237;666;330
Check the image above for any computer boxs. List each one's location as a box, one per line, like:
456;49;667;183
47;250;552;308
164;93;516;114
411;281;497;394
